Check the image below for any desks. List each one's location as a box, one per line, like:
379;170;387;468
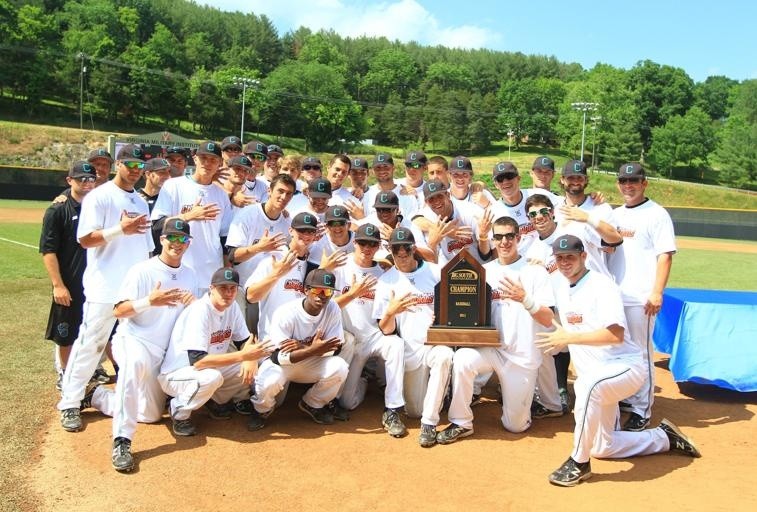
651;287;756;394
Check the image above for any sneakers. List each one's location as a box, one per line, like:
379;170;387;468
298;398;351;425
382;408;406;438
112;437;134;472
167;401;194;436
548;456;592;487
531;387;569;419
205;399;276;431
619;401;650;432
57;368;111;410
419;423;474;447
658;417;700;458
61;408;83;432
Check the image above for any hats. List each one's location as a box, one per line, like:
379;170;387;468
552;234;583;254
68;143;188;179
373;190;398;208
291;205;349;228
355;223;414;244
305;269;335;288
423;178;447;200
303;157;332;199
493;156;586;177
619;162;645;178
450;155;472;172
164;218;194;239
211;267;240;286
350;152;427;170
197;136;283;170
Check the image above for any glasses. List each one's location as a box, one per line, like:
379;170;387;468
619;178;639;184
295;229;316;233
493;233;516;240
311;288;334;297
529;207;551;218
166;235;188;243
393;244;411;250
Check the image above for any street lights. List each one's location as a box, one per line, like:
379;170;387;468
588;113;600;171
570;102;599;164
230;77;260;144
506;129;514;160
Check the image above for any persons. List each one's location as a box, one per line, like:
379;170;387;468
39;133;703;487
